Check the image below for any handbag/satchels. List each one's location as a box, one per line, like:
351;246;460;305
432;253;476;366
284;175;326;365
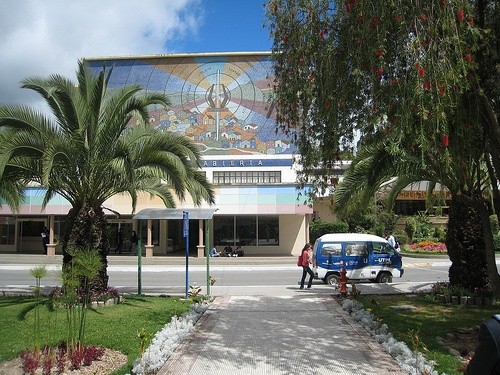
298;255;303;266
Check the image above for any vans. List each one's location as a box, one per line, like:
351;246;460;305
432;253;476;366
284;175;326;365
311;232;404;287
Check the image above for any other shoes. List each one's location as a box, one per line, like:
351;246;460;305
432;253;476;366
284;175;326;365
306;287;313;289
300;287;306;289
400;269;404;278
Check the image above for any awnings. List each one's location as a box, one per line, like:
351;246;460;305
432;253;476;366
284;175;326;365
132;208;220;295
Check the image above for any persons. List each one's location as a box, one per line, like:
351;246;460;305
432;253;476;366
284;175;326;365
210;243;221;257
300;243;314;289
114;228;123;254
233;246;243;257
384;228;404;277
129;231;137;256
223;243;232;257
41;227;49;255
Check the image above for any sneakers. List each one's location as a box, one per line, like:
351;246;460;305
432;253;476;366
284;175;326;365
233;254;238;257
228;254;231;257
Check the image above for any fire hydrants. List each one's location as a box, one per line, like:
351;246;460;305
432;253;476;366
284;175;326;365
337;267;349;298
